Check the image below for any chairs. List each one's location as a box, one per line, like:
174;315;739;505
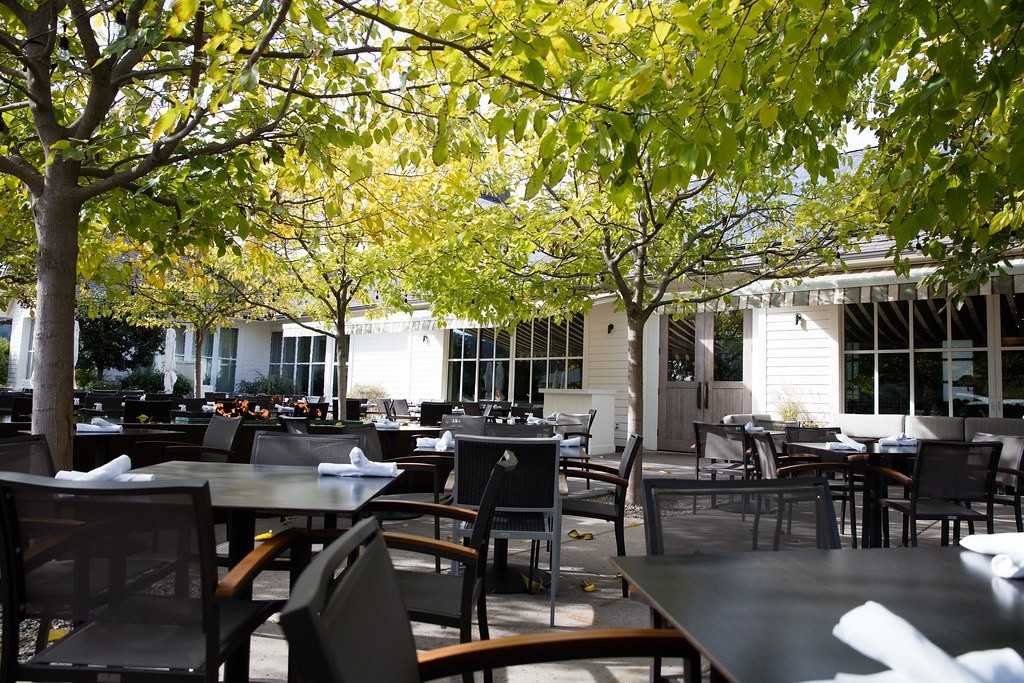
0;391;1024;683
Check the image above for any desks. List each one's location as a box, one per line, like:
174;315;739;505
485;420;583;427
363;422;455;521
15;428;187;473
787;442;984;555
414;447;553;596
131;460;407;683
510;403;546;409
610;552;1024;683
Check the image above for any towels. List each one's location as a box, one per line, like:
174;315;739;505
361;401;377;408
825;434;867;452
879;433;917;446
275;404;294;412
527;417;546;425
736;422;764;432
55;454;155;497
318;447;397;477
959;533;1024;579
416;430;455;451
375;419;400;429
832;600;1024;683
202;402;215;412
76;418;123;432
298;397;306;403
552;434;580;446
